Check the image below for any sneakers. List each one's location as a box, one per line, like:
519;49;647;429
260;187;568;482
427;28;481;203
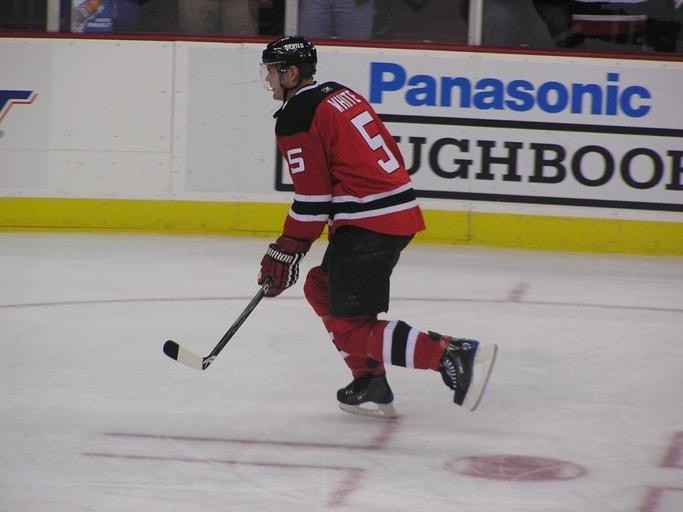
338;373;394;405
440;337;479;405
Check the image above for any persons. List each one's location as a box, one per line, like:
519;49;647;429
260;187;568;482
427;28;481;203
257;35;497;418
177;1;259;37
299;0;374;41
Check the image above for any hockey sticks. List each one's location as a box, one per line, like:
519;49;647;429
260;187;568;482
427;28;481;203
164;277;274;370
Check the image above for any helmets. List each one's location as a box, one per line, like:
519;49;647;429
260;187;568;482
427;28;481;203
259;35;317;90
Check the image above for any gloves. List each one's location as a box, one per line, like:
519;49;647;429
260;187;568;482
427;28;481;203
257;235;311;298
